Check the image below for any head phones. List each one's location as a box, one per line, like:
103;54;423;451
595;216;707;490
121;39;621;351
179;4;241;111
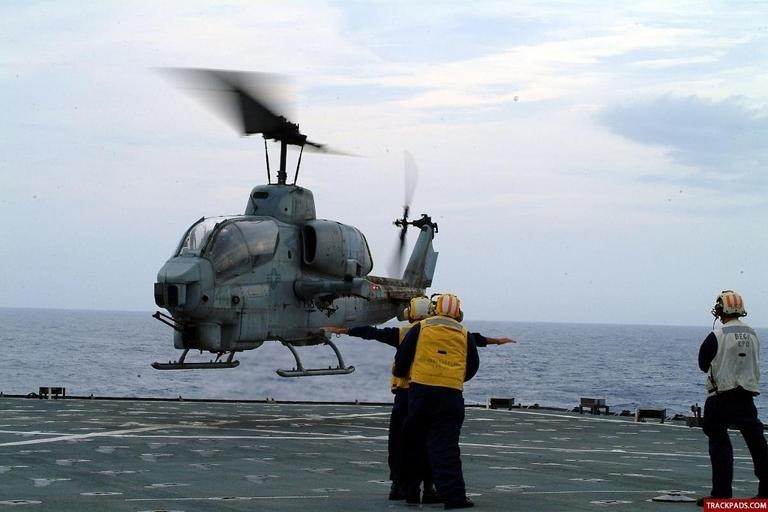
715;304;747;317
429;293;463;322
403;295;429;320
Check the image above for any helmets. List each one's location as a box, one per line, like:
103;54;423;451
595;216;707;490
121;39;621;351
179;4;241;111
410;294;460;319
716;290;744;316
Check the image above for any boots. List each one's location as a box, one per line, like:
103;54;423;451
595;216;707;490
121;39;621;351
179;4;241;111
749;481;767;499
696;487;733;507
388;488;474;510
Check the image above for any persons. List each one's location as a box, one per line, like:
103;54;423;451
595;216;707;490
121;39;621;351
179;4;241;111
696;289;768;507
320;293;517;510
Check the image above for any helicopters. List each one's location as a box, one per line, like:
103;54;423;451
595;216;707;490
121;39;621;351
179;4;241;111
134;63;440;378
